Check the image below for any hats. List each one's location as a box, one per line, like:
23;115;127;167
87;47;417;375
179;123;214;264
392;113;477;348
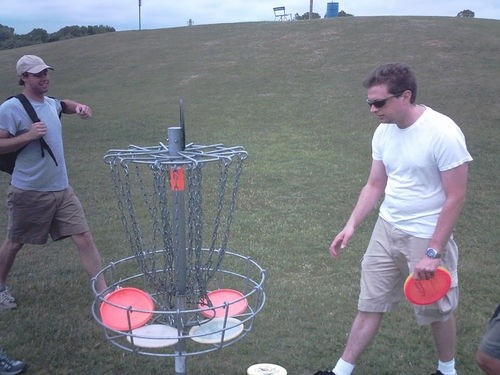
16;54;54;76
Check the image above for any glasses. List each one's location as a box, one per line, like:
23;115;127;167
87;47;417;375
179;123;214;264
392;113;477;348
366;92;401;109
34;69;48;77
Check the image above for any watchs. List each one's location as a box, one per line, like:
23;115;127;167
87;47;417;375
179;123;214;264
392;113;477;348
424;248;441;258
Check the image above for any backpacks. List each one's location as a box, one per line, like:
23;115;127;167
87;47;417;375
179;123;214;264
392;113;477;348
0;93;58;176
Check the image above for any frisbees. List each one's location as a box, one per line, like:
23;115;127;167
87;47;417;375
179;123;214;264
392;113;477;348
198;289;248;318
403;266;452;305
101;287;154;331
126;324;178;348
189;317;244;344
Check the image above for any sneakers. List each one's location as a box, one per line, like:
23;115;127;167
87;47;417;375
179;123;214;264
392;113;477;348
0;351;27;375
0;285;17;306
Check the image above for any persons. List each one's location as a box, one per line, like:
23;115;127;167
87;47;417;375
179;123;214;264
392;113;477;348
0;55;110;310
0;353;26;375
313;63;474;375
475;303;500;375
